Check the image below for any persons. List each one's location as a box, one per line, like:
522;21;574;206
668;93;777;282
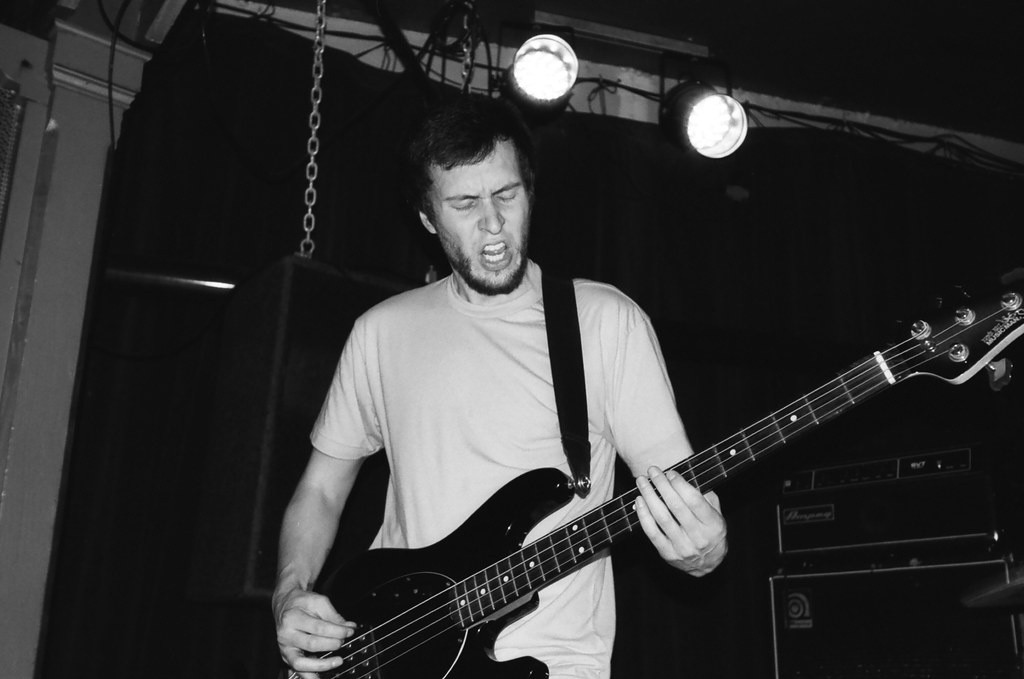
269;99;729;679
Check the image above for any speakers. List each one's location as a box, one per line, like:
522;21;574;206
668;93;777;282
767;448;1024;676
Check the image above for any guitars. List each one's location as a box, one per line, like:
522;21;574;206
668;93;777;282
280;291;1024;679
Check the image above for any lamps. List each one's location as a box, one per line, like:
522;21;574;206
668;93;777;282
509;33;580;115
658;76;748;159
